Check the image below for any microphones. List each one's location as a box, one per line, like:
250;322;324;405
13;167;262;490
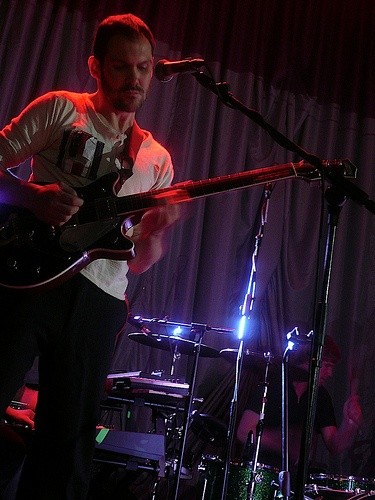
127;312;151;333
153;58;205;82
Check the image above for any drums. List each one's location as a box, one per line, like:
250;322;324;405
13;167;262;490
195;453;282;500
304;472;375;500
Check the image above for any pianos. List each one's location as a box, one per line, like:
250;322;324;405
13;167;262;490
3;410;167;469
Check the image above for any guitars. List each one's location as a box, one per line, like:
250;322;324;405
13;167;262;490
1;154;358;295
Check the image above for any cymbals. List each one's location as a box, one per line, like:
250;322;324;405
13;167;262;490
126;331;221;358
219;348;284;369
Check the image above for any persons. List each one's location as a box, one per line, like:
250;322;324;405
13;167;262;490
0;14;180;500
236;333;359;472
